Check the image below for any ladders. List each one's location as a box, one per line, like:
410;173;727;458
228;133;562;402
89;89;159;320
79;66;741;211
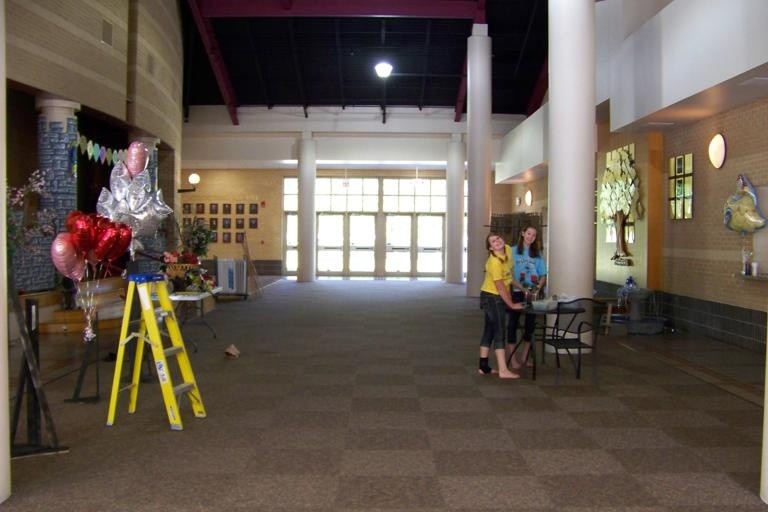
106;272;206;431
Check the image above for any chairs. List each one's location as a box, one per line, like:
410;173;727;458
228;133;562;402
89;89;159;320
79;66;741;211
530;298;604;381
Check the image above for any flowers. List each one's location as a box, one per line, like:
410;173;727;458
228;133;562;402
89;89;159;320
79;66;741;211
519;273;540;296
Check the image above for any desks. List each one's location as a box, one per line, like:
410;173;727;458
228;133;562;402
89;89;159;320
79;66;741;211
504;301;586;368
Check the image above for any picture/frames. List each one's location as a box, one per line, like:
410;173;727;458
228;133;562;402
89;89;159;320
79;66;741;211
181;203;258;244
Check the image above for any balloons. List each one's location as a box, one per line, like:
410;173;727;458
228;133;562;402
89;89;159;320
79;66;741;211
51;141;173;282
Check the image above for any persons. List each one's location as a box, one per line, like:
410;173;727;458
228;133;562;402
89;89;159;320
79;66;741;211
479;224;547;379
